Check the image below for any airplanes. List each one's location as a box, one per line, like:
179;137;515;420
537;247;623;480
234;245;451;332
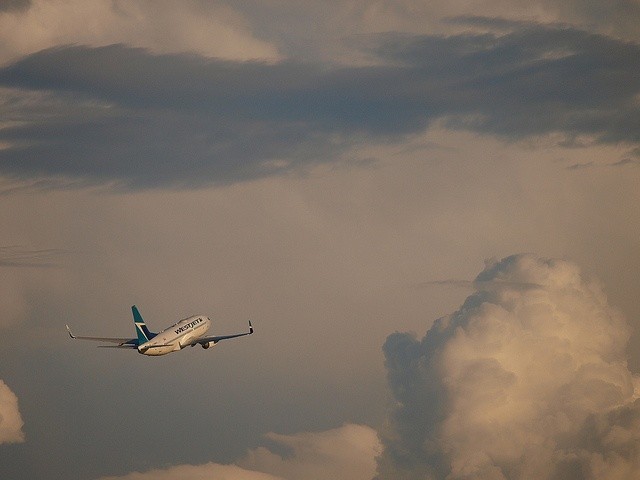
65;305;254;356
